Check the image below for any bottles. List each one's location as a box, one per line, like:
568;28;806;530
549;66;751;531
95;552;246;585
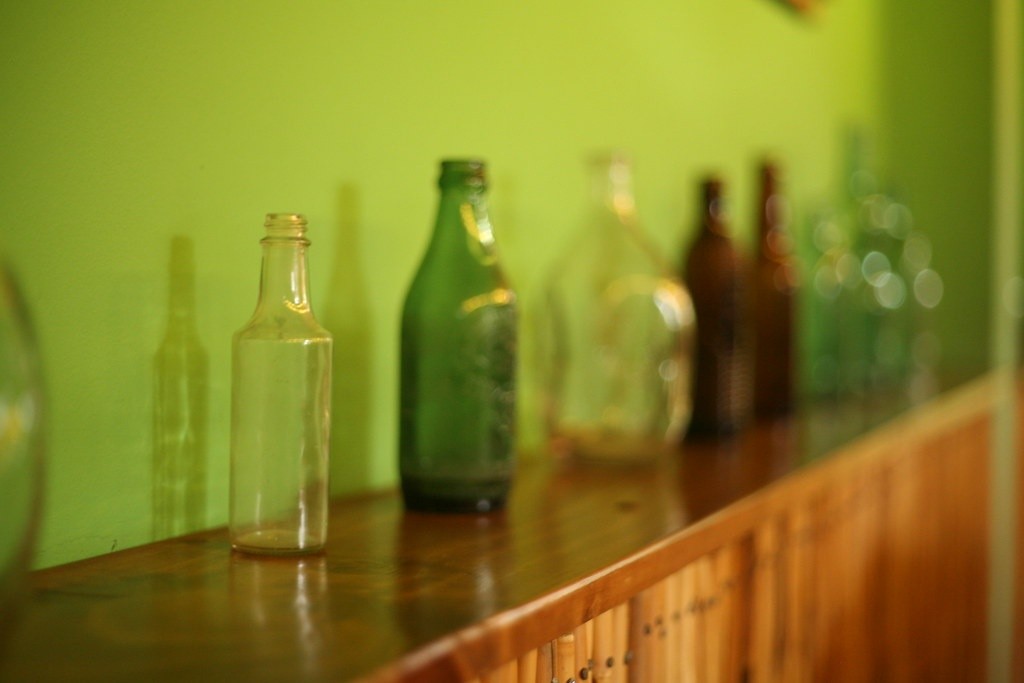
397;157;519;513
546;155;696;463
0;263;48;634
681;180;748;440
749;160;798;420
230;215;333;554
